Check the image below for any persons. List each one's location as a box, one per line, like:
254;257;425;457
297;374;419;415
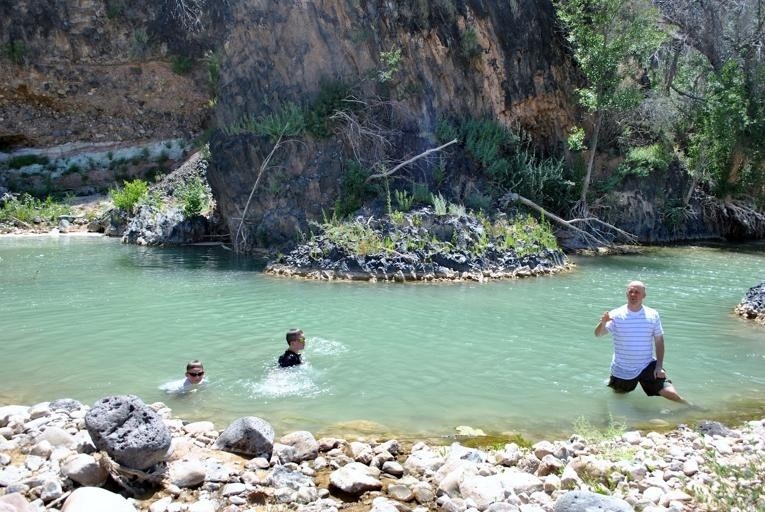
278;328;306;367
175;360;208;399
591;280;709;413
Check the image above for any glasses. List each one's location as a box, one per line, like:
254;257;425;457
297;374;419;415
294;337;306;343
187;372;204;377
